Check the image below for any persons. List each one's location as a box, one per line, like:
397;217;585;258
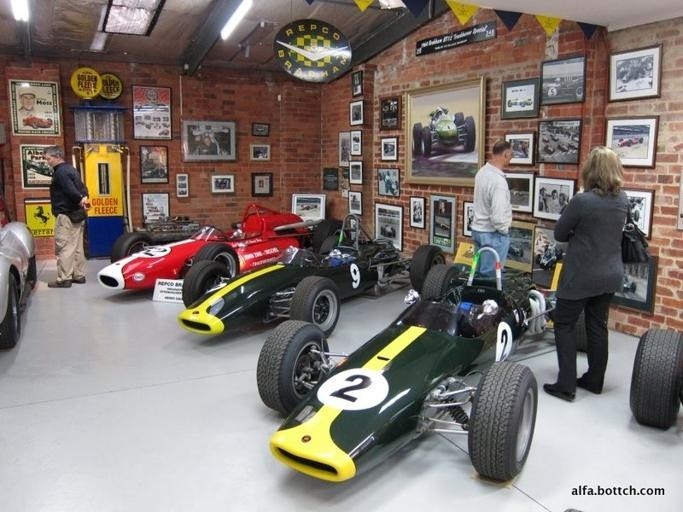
16;88;38;114
433;107;447;121
539;187;568;217
142;151;165;177
43;147;92;288
543;147;650;403
470;140;514;273
199;137;217;155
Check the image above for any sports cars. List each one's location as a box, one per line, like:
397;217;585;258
0;220;37;348
23;116;52;128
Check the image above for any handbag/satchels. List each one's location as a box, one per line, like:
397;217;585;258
621;205;649;265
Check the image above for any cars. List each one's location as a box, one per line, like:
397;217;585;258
256;246;590;485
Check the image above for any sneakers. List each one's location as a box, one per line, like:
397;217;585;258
72;276;86;284
47;280;72;288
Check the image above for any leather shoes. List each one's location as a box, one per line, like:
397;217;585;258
543;383;575;402
576;377;602;395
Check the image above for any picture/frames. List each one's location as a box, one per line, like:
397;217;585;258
377;168;401;198
174;172;189;199
535;116;582;167
606;42;662;103
503;131;535;165
7;79;61;137
609;255;657;318
349;130;361;156
131;85;172;141
603;114;660;168
380;136;398;162
428;193;456;256
209;173;235;195
616;187;655;241
19;144;58;190
251;122;270;137
349;71;362;98
403;75;486;188
23;197;54;237
347;190;363;216
348;161;362;185
532;175;577;221
249;144;271;161
140;192;170;221
501;169;535;212
349;100;363;126
178;119;237;163
251;172;273;197
503;218;537;273
138;144;169;184
377;95;402;131
322;167;338;191
500;77;540;120
531;225;570;291
337;131;351;167
409;196;426;230
291;194;325;223
373;202;404;252
540;55;585;107
462;200;473;237
